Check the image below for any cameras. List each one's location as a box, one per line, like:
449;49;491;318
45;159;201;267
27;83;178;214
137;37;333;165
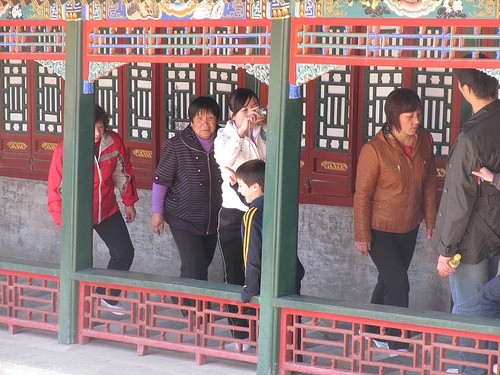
255;107;267;119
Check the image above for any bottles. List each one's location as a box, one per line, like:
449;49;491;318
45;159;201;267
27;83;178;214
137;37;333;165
448;254;462;268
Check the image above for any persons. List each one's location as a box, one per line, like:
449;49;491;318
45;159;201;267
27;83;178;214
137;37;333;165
151;95;222;323
46;104;139;316
225;159;305;362
471;167;500;375
214;88;268;350
436;53;500;375
355;87;437;358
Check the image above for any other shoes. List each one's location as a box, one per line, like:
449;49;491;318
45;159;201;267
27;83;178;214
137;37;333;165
446;368;458;373
358;330;390;350
101;299;126;315
235;337;250;351
390;348;409;358
492;364;497;374
172;295;191;318
